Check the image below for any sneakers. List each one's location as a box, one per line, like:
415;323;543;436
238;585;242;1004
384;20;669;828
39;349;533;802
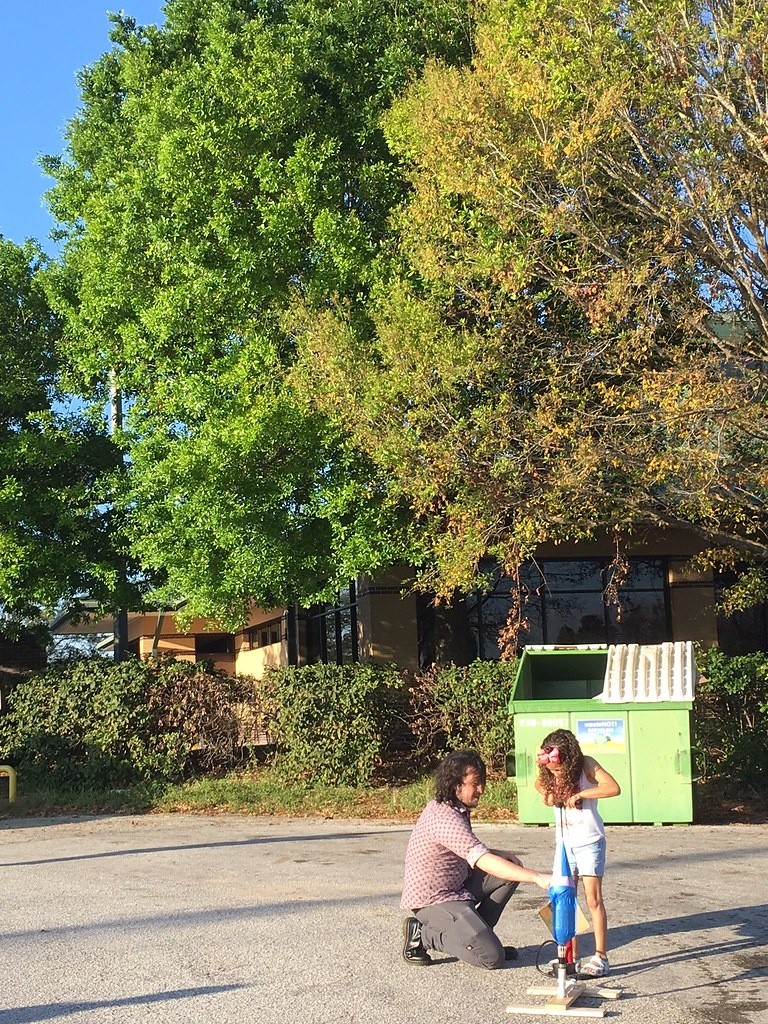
402;917;432;965
503;947;518;959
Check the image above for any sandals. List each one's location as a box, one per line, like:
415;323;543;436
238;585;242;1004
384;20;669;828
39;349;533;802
544;958;559;970
580;955;610;976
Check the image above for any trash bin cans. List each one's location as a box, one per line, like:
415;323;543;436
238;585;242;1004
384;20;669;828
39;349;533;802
508;641;698;828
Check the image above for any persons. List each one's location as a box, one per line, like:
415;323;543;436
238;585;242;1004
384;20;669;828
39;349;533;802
534;729;621;980
399;748;550;971
